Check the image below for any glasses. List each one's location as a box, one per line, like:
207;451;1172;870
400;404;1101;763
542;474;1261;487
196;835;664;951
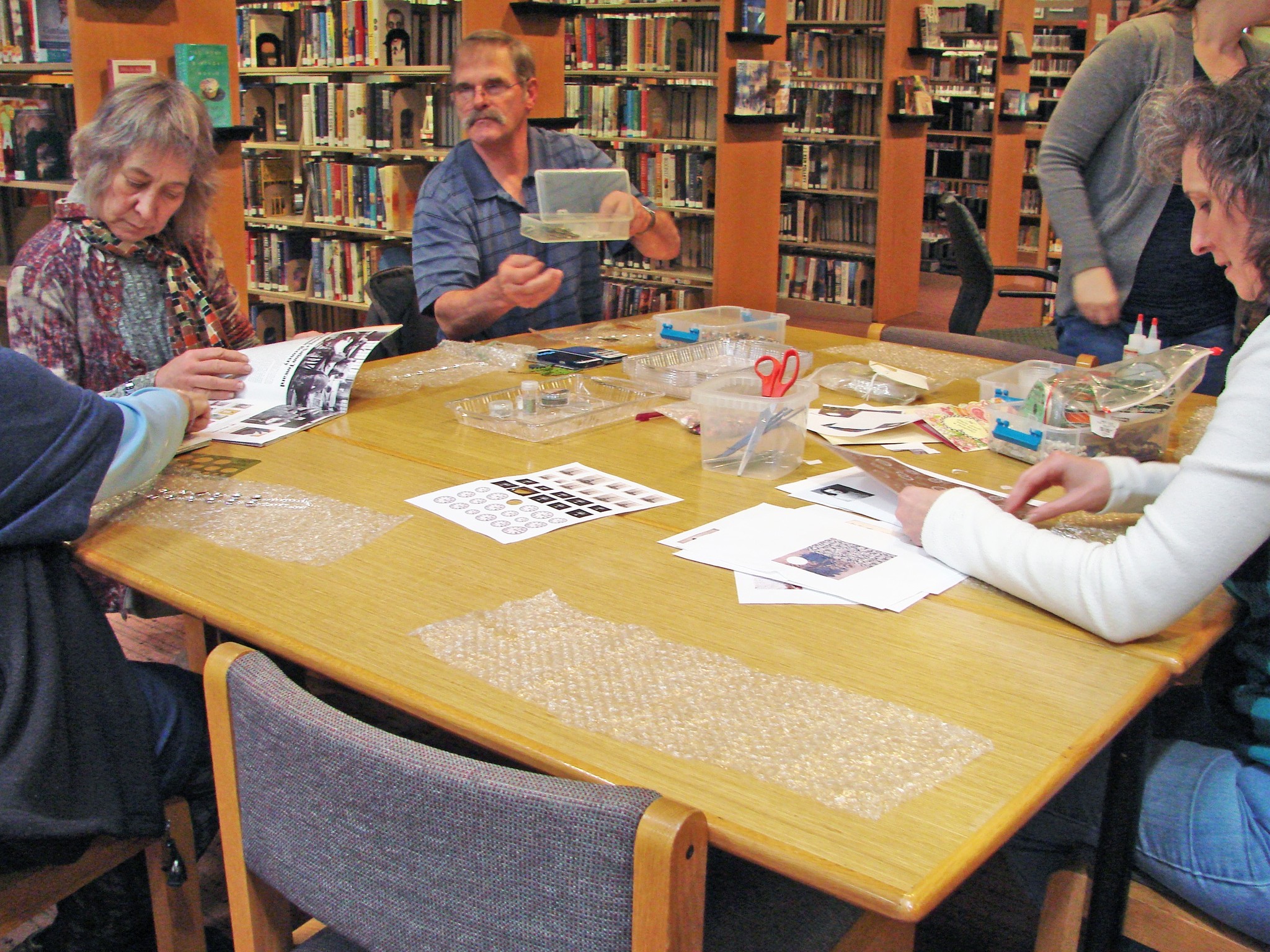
447;77;528;104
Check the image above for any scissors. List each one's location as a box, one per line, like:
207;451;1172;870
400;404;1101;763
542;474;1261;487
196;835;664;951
737;348;801;477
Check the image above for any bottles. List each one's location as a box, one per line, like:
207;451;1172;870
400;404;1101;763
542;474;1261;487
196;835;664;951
1122;314;1147;360
1137;318;1162;356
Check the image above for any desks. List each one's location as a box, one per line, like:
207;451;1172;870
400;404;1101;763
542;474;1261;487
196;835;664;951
68;305;1237;926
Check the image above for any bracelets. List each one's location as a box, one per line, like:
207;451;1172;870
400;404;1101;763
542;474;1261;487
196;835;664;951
634;205;655;236
124;378;134;397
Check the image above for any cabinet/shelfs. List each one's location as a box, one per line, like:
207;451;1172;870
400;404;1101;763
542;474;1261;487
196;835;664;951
0;1;1197;343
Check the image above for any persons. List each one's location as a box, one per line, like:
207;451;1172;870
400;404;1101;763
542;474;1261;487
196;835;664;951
413;28;679;346
0;344;221;952
895;62;1270;948
1036;0;1270;395
7;75;331;620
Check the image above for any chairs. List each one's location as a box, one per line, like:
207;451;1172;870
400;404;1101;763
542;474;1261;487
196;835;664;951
934;188;1061;352
866;322;1099;369
366;264;443;356
200;634;920;952
0;796;207;952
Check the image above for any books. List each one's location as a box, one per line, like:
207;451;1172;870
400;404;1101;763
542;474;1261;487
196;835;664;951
733;0;885;310
236;0;461;346
0;0;71;65
5;187;68;265
898;0;1090;329
107;58;157;94
0;71;77;182
564;8;721;319
174;43;232;128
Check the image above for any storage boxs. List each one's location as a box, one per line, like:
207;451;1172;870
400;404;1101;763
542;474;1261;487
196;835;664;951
518;167;636;245
690;372;822;484
987;399;1176;466
974;359;1089;405
652;303;792;352
